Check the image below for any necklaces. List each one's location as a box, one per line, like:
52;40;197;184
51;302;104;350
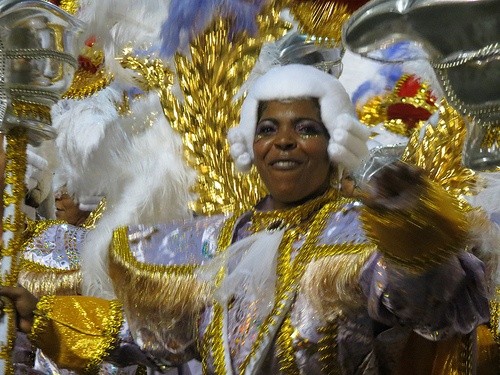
247;185;337;236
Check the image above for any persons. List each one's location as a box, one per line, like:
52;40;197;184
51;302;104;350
1;180;108;375
1;58;491;375
